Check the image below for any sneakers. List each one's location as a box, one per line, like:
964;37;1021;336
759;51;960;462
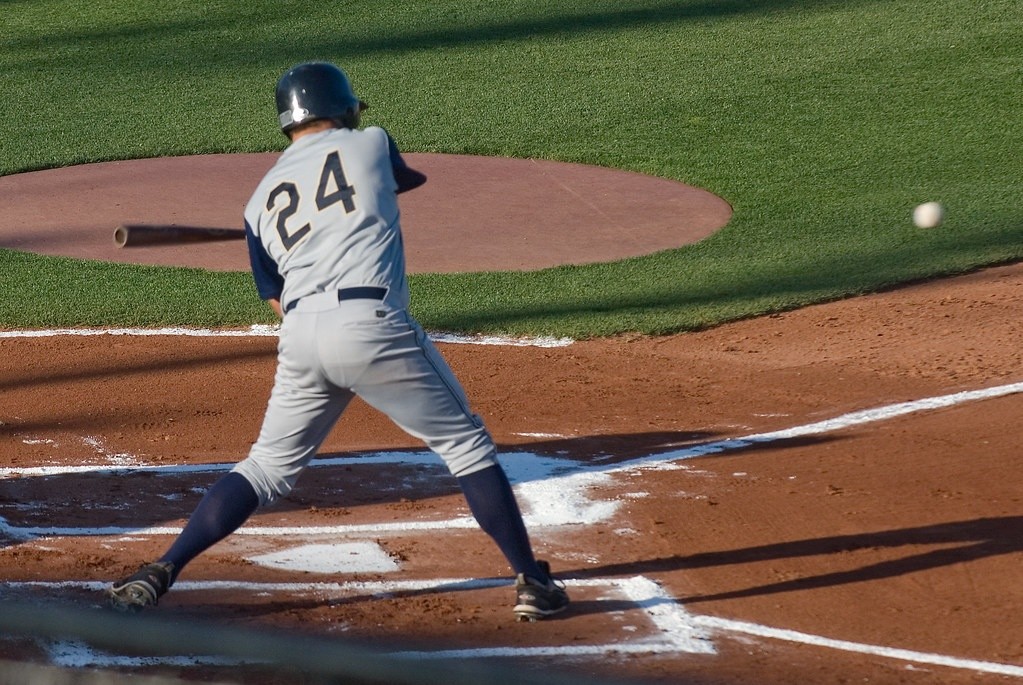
110;561;176;614
513;559;570;621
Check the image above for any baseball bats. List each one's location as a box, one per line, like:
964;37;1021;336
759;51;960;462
112;222;248;250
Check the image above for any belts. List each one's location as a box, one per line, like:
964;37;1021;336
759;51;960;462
286;286;388;310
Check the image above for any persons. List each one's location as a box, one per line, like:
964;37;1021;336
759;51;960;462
109;62;570;623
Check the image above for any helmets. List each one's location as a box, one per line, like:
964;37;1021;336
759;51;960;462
276;63;370;131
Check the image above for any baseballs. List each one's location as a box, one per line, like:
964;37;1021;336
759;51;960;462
911;201;944;229
